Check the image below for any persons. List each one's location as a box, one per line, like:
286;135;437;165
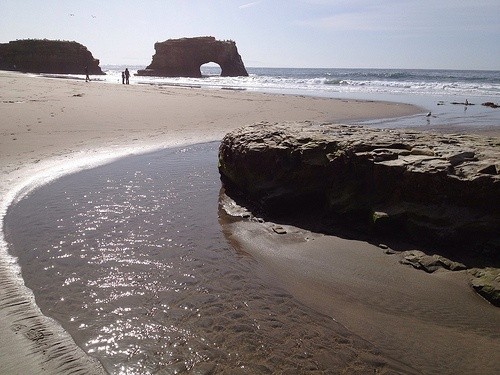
122;72;125;84
82;64;91;82
125;68;129;84
